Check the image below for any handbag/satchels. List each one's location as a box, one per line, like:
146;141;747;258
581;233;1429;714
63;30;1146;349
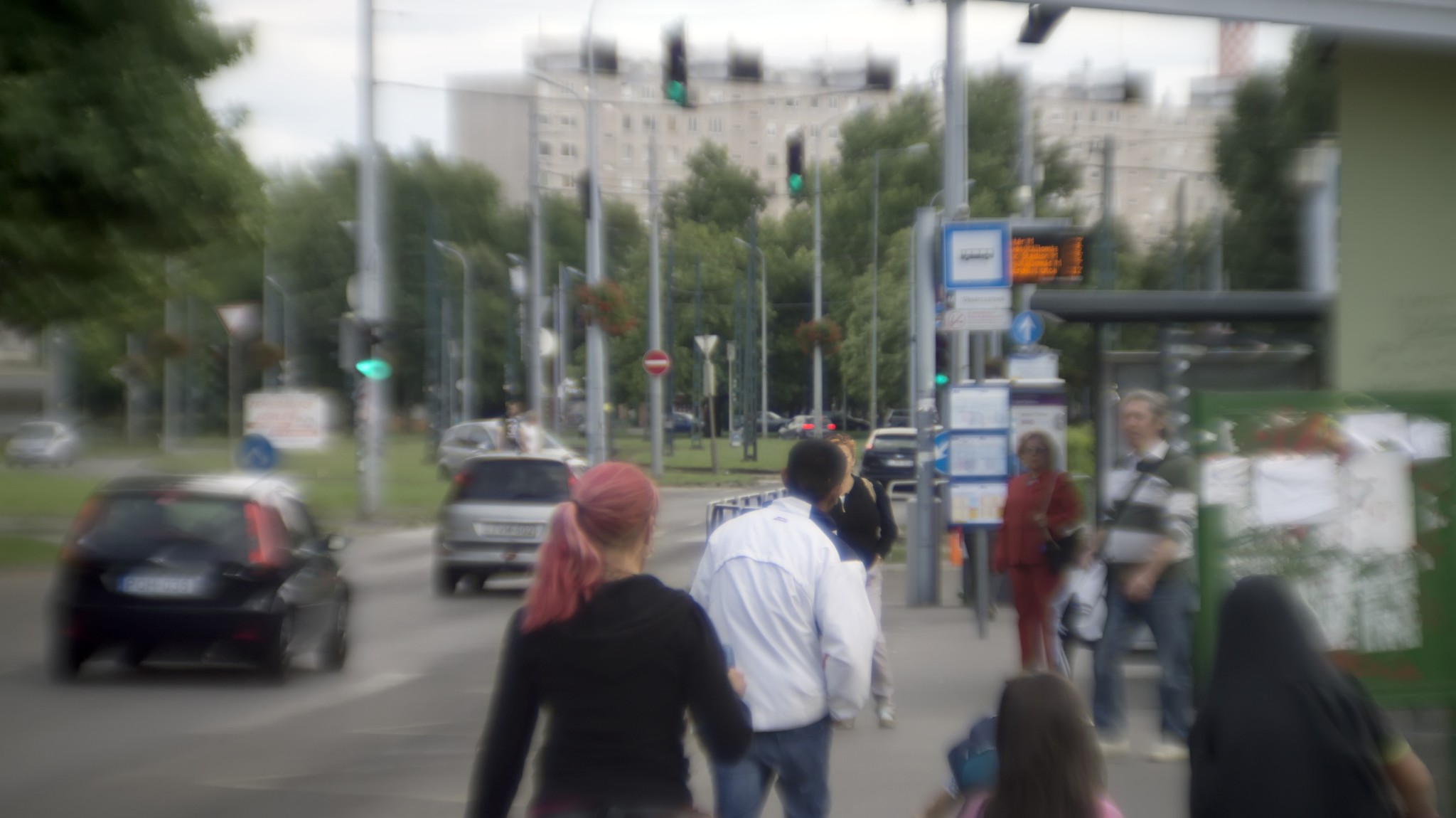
1061;553;1109;641
1046;532;1084;569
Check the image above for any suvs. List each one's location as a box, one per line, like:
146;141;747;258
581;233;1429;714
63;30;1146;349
809;409;872;431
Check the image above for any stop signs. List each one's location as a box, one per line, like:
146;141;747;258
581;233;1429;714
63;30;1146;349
641;348;670;376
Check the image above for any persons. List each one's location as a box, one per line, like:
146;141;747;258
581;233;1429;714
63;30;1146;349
496;400;531;453
987;430;1087;685
1087;390;1204;763
464;462;756;818
1188;573;1441;818
691;434;897;818
918;669;1125;818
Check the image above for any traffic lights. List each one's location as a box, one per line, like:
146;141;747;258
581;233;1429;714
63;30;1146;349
661;33;688;105
786;135;807;200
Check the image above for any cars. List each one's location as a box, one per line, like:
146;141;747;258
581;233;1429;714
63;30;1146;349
777;414;837;438
41;469;355;688
674;412;705;431
755;411;790;431
2;419;81;465
431;447;592;597
860;407;919;494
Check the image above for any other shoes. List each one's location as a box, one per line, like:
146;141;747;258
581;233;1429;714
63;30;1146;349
1150;743;1191;762
876;705;897;728
836;719;856;731
1100;737;1131;758
